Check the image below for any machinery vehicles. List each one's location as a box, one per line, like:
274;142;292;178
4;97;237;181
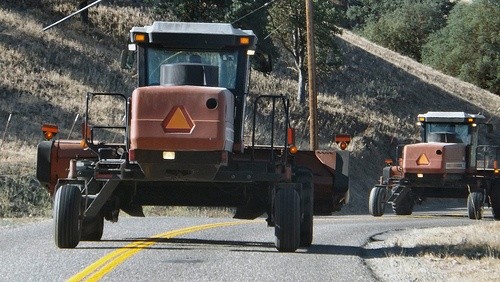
35;20;354;252
368;111;500;220
